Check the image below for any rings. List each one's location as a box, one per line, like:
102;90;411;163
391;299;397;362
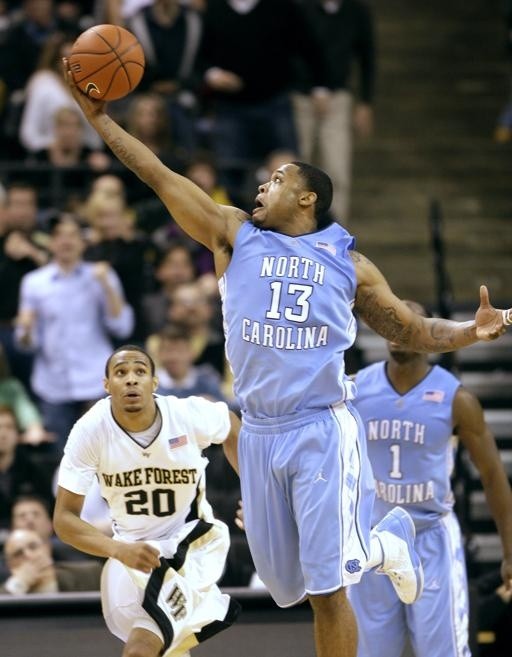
506;307;512;323
501;309;511;326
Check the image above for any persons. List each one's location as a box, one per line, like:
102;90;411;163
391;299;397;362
48;343;246;657
1;1;379;599
56;50;512;657
346;296;512;657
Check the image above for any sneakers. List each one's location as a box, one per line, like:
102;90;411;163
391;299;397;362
370;506;424;605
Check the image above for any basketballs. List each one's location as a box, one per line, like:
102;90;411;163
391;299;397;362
70;24;145;104
